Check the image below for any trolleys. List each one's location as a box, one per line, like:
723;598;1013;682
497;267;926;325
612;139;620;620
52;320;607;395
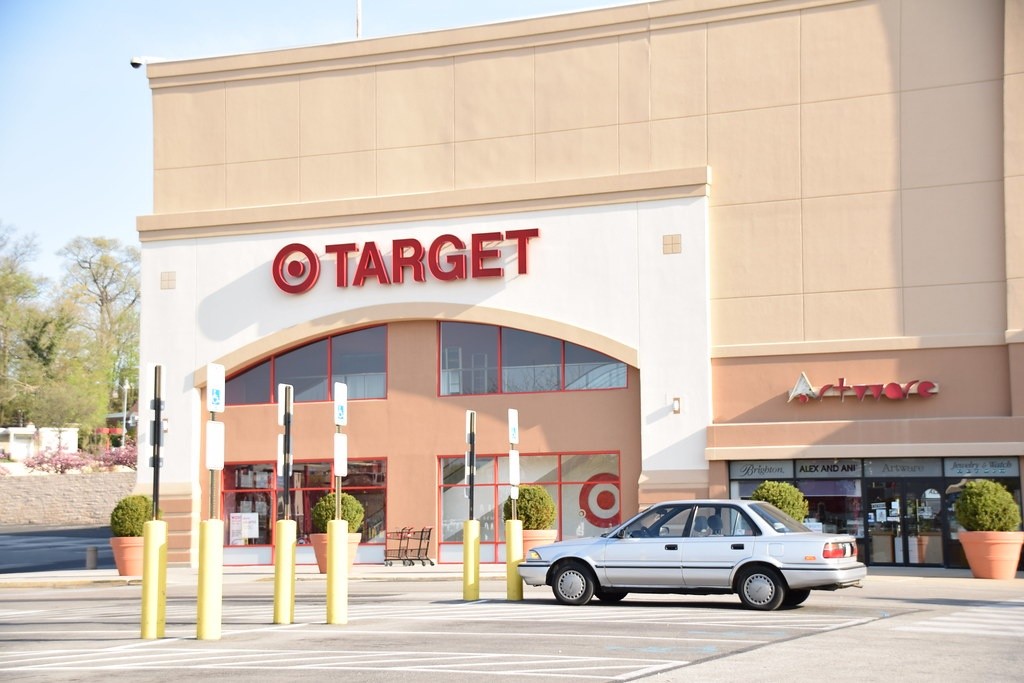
384;528;435;567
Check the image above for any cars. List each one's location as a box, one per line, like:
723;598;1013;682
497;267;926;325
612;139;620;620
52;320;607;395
919;511;937;520
517;499;868;611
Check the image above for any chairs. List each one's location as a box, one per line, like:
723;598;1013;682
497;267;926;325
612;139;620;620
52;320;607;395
691;516;708;537
707;515;724;536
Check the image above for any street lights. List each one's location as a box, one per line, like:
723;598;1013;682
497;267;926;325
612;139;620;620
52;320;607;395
97;380;127;448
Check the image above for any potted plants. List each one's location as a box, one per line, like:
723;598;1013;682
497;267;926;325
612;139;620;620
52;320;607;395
503;484;558;558
108;494;164;576
309;492;366;573
955;480;1024;579
